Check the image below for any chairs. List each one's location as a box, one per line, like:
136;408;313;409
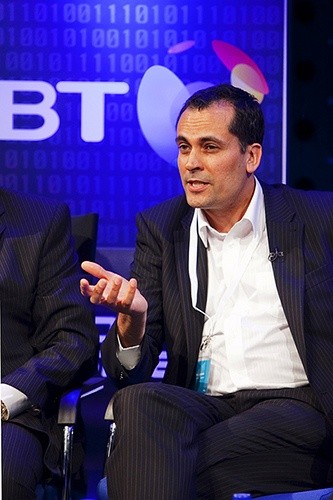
49;213;106;500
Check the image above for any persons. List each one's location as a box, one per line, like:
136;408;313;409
0;184;101;500
76;86;332;499
